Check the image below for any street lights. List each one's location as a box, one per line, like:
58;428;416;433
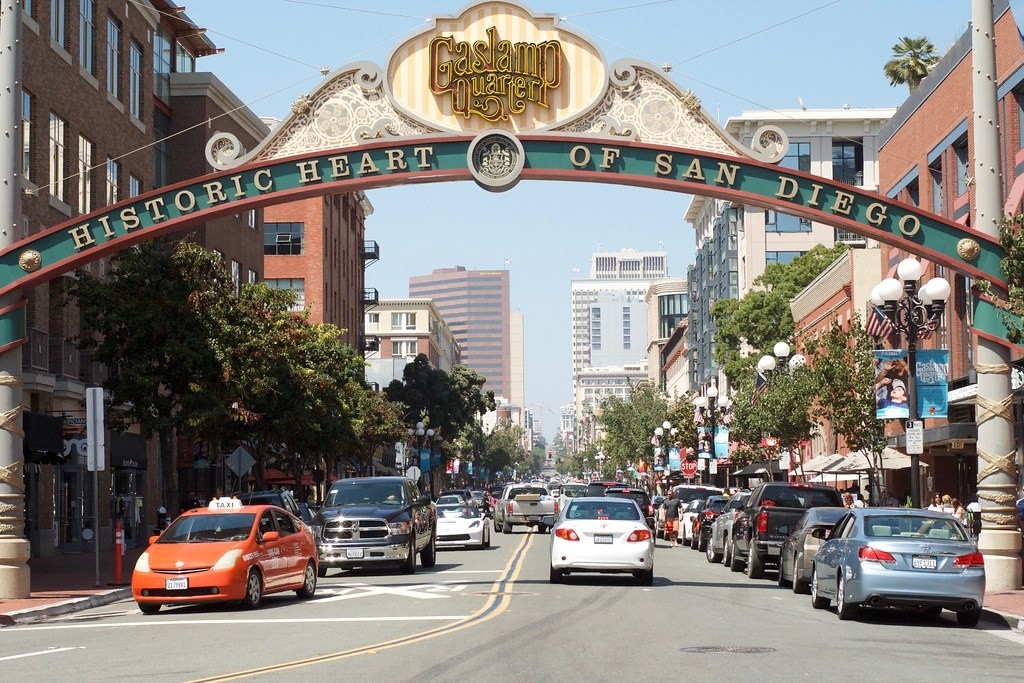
872;257;953;507
408;422;434;478
758;341;805;481
695;387;728;485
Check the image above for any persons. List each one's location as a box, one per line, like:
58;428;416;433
414;508;436;535
654;455;666;468
661;490;684;547
837;483;1024;586
698;433;714;458
179;487;404;515
873;354;910;418
437;491;499;521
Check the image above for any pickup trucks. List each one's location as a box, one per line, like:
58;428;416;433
729;484;846;578
494;483;558;533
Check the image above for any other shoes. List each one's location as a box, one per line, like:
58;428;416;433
671;544;673;546
675;544;678;546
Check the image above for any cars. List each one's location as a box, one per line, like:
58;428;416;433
679;495;728;550
776;507;986;627
542;497;656;585
433;504;493;550
434;489;484;507
130;506;320;612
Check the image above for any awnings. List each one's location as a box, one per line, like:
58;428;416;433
339;451;401;477
728;461;785;479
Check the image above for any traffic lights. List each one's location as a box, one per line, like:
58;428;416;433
549;452;551;458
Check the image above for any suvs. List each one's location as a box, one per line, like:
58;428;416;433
309;475;437;577
232;488;302;521
602;486;655;529
705;489;754;567
577;482;630;497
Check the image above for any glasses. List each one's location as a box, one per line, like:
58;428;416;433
666;494;671;495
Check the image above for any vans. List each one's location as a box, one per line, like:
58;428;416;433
656;483;721;538
554;483;588;514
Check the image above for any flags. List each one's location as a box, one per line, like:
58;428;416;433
752;371;770;406
912;311;939;340
866;305;898;340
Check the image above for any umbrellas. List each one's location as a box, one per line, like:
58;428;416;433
241;467;342;488
788;445;928;508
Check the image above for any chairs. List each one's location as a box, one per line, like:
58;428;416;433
811;495;832;507
574;510;592;519
928;529;950;540
264;520;291;532
866;525;892;537
777;494;801;508
615;511;631;520
